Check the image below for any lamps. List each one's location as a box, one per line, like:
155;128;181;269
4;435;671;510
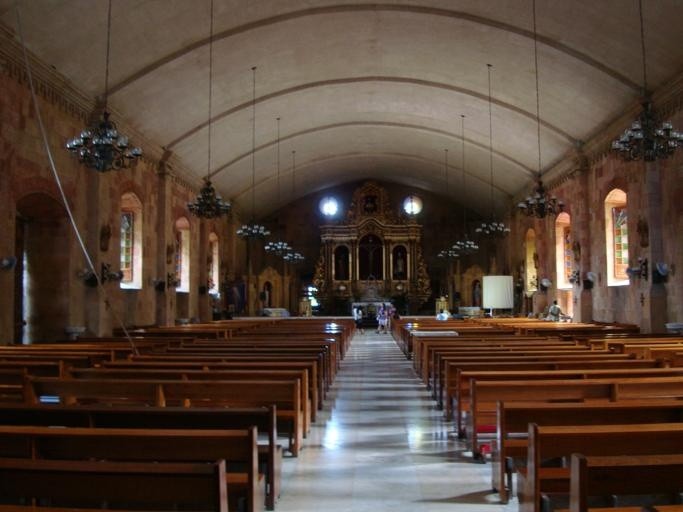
63;0;144;172
264;115;293;257
513;0;566;218
396;190;425;217
435;149;460;261
186;0;234;219
473;65;512;239
282;150;306;266
451;114;481;254
234;66;271;247
316;193;340;217
608;0;683;163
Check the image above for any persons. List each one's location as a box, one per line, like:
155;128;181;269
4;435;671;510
550;301;565;321
436;309;448;321
388;303;397;331
375;303;388;334
356;306;365;334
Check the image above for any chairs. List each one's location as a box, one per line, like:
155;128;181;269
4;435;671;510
0;314;358;512
388;312;682;511
